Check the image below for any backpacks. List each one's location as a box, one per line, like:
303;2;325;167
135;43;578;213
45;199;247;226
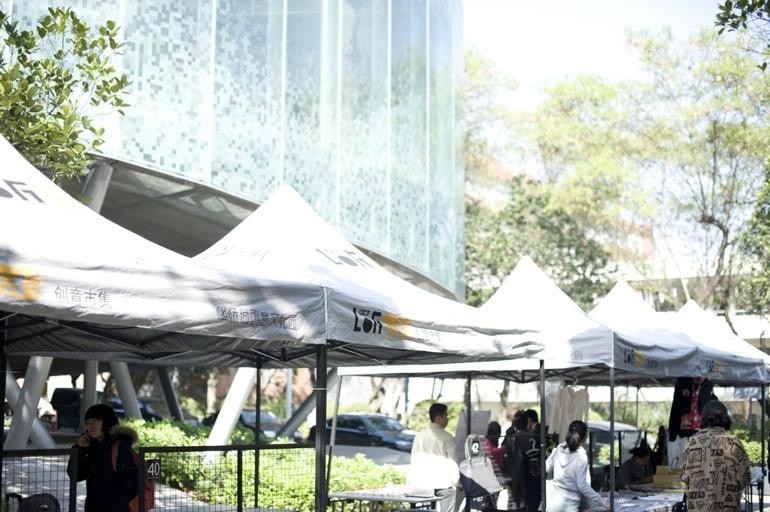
110;443;154;510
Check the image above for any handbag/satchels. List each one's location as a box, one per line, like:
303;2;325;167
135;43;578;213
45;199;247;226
460;456;503;499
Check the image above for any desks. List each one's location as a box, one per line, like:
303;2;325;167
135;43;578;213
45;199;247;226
329;484;453;512
577;453;770;512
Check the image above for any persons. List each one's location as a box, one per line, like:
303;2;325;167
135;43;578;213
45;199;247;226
679;400;752;512
459;433;503;512
67;403;140;512
483;420;507;476
504;413;541;511
506;410;530;437
410;403;467;512
615;447;654;491
537;419;610;512
506;408;539;436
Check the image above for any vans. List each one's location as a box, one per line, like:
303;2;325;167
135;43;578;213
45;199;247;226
52;387;104;433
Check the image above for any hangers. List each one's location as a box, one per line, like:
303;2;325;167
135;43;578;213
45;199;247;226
522;438;556;457
560;373;587;394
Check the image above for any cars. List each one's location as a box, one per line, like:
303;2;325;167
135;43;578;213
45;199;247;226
586;419;659;461
309;411;419;453
4;398;14;416
111;397;198;432
203;408;305;444
